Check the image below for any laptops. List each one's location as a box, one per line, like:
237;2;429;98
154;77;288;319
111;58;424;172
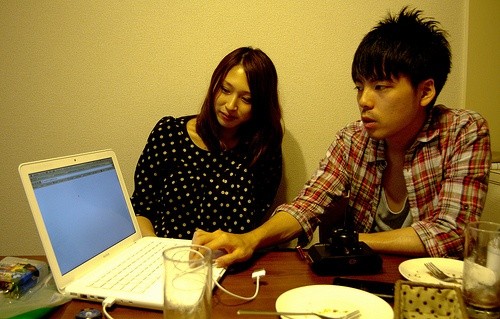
18;149;232;311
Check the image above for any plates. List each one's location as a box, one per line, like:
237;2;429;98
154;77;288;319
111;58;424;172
398;257;497;291
275;284;395;319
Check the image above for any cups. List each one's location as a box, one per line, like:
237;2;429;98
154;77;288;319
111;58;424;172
462;223;500;319
162;244;214;319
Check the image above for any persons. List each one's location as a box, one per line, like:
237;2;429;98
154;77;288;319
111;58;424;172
188;5;493;268
130;46;284;240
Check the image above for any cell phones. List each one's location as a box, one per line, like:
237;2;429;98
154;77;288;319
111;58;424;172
332;277;395;302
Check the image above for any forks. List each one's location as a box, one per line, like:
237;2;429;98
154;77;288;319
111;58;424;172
424;262;463;281
236;309;361;319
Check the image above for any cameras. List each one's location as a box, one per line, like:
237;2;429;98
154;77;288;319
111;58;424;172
305;224;384;276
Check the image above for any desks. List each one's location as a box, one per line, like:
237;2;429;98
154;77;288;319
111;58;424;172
0;250;421;319
